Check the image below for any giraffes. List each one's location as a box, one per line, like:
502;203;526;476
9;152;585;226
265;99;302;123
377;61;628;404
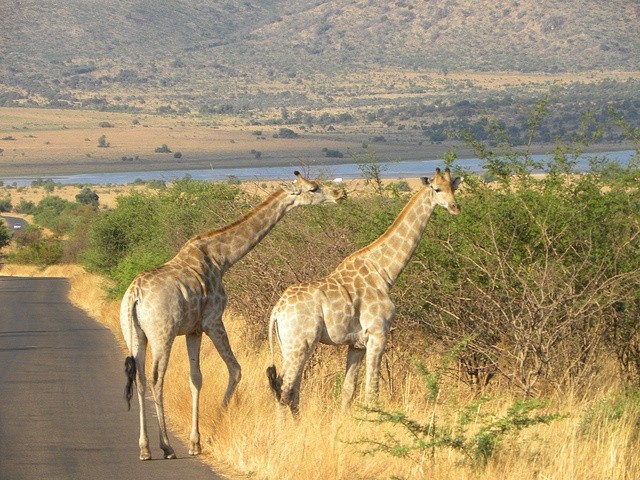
267;168;465;435
120;170;347;461
